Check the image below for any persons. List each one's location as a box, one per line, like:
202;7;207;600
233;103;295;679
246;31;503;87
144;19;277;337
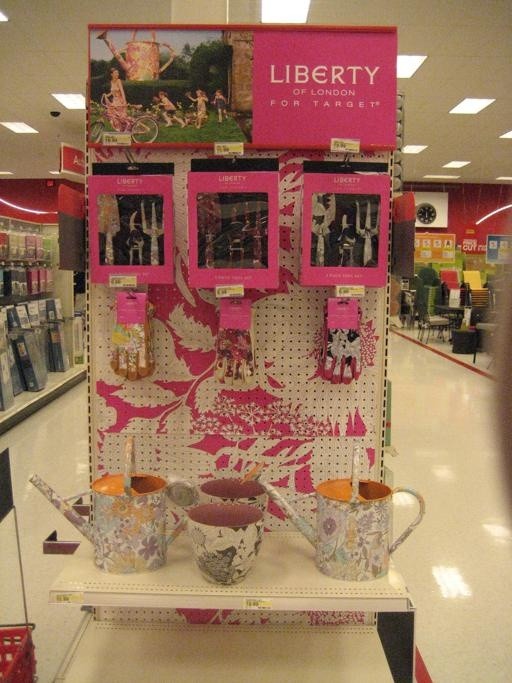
212;90;228;123
187;90;208;129
105;68;131;132
156;91;185;129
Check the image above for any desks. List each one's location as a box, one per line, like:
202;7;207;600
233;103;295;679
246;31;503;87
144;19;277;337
431;305;484;343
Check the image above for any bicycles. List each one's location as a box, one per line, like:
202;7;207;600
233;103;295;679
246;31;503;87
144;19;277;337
90;93;159;144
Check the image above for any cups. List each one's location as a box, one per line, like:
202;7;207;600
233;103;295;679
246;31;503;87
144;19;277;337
189;503;264;585
201;480;268;514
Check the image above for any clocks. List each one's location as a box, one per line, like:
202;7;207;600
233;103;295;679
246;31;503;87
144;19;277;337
413;193;448;227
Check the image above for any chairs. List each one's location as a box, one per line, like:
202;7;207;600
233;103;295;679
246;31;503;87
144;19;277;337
439;270;460;306
463;271;489;309
417;288;448;344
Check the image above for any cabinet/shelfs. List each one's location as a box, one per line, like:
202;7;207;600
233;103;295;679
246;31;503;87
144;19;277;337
1;218;88;436
43;130;429;683
